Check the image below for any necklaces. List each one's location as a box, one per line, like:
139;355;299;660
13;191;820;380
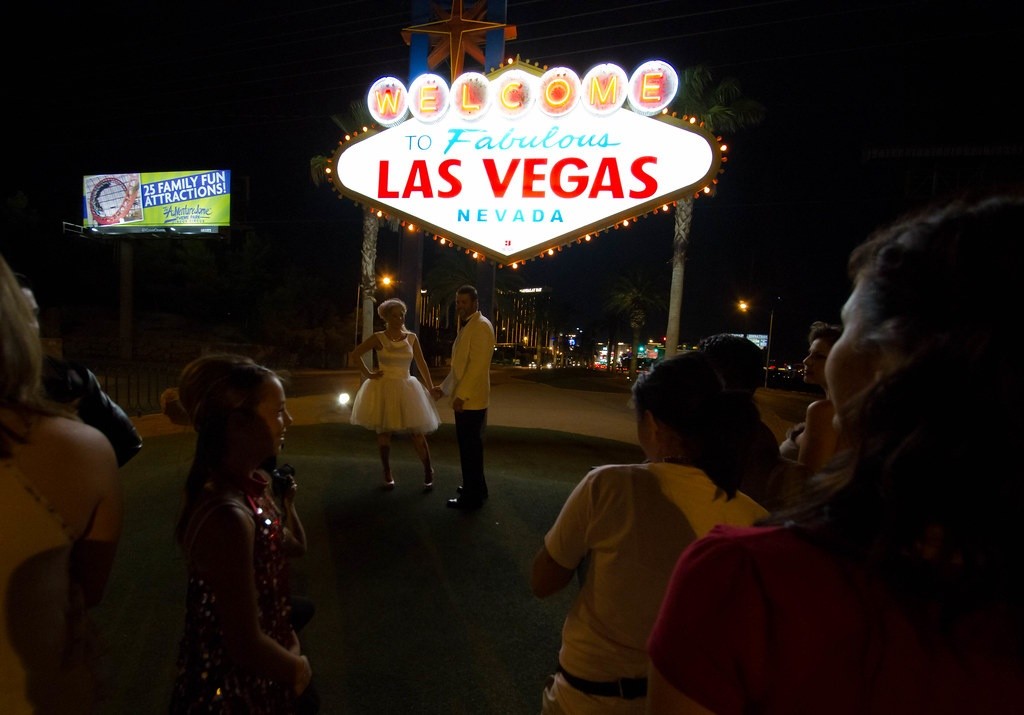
385;330;404;342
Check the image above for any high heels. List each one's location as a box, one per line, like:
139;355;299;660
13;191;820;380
381;469;395;489
423;466;434;490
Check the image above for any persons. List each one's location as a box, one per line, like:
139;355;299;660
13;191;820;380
432;286;495;509
1;255;143;715
779;320;845;469
646;194;1024;715
684;336;780;510
351;299;440;489
160;354;310;715
185;367;314;714
530;353;774;715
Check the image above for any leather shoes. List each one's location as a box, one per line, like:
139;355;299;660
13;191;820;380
449;498;480;512
457;485;488;500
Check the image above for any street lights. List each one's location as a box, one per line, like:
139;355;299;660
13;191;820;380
419;289;428;326
